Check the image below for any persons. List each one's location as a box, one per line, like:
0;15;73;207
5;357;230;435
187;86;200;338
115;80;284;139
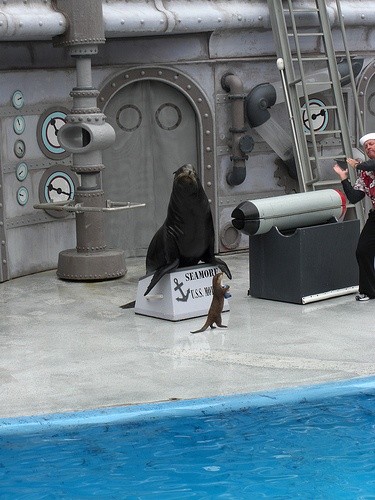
334;132;375;303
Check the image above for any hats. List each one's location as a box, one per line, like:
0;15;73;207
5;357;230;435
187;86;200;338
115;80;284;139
359;132;375;146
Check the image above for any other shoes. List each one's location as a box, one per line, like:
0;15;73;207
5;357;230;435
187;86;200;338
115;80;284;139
355;292;370;301
